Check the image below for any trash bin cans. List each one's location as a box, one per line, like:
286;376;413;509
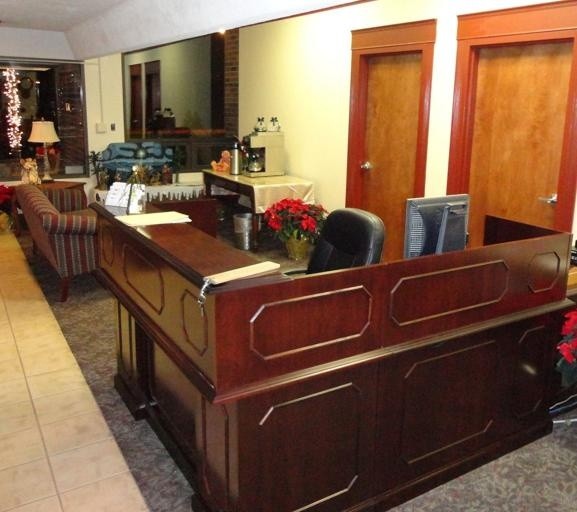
233;213;263;250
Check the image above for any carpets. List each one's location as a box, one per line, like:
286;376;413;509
8;212;577;511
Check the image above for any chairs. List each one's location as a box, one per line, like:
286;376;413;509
14;184;97;302
283;208;385;276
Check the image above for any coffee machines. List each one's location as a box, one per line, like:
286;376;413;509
240;132;286;177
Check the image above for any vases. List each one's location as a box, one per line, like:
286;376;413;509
284;237;310;260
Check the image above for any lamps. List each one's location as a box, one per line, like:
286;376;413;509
26;121;61;182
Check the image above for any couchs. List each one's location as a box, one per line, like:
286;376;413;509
96;142;174;186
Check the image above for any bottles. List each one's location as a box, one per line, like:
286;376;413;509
267;116;282;132
151;107;176;121
229;141;243;176
253;116;268;132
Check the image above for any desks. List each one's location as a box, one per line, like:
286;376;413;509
203;169;312;254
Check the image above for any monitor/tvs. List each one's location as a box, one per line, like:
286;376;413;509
402;193;470;258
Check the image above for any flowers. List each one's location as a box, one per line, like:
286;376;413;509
262;196;330;245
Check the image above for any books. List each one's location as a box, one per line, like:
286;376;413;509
241;170;285;178
